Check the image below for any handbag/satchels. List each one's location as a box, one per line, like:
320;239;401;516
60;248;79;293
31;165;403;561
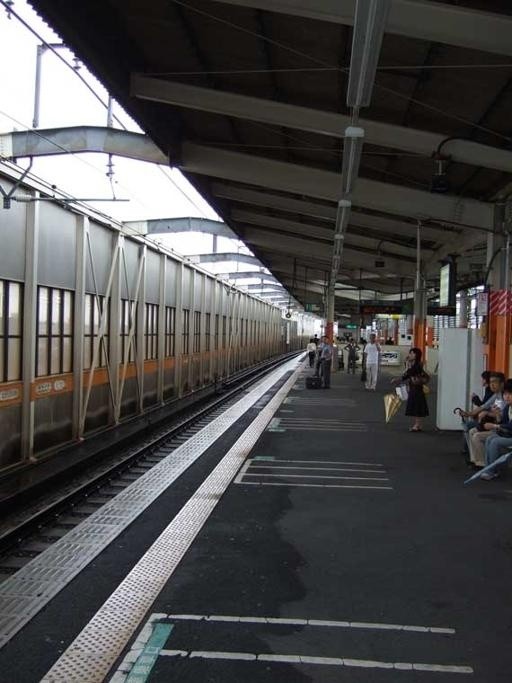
410;370;430;386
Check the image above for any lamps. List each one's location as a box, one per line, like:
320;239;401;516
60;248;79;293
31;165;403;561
328;0;393;256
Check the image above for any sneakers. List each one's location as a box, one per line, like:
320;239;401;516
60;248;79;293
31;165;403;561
408;425;424;432
463;456;500;482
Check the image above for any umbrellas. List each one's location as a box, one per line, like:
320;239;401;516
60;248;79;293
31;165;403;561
384;393;402;423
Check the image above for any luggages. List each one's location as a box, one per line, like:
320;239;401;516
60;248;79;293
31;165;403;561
305;377;323;389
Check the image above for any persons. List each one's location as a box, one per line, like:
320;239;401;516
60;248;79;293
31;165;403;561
456;367;512;481
318;335;333;390
361;333;382;393
385;336;394;344
306;332;361;375
390;346;429;432
